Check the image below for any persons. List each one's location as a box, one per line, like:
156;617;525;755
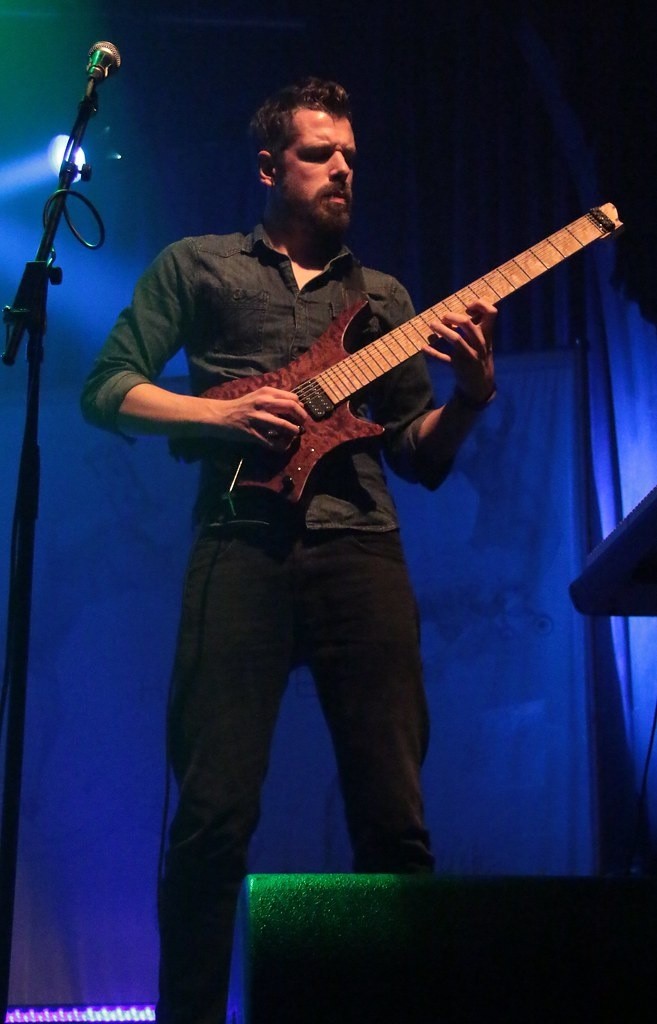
79;72;500;1024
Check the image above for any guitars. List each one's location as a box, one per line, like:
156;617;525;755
168;201;626;511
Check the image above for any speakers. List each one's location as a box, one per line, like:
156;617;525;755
226;873;657;1024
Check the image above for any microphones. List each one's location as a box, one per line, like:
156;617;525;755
87;40;121;82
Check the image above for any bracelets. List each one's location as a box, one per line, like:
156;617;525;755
454;378;497;411
